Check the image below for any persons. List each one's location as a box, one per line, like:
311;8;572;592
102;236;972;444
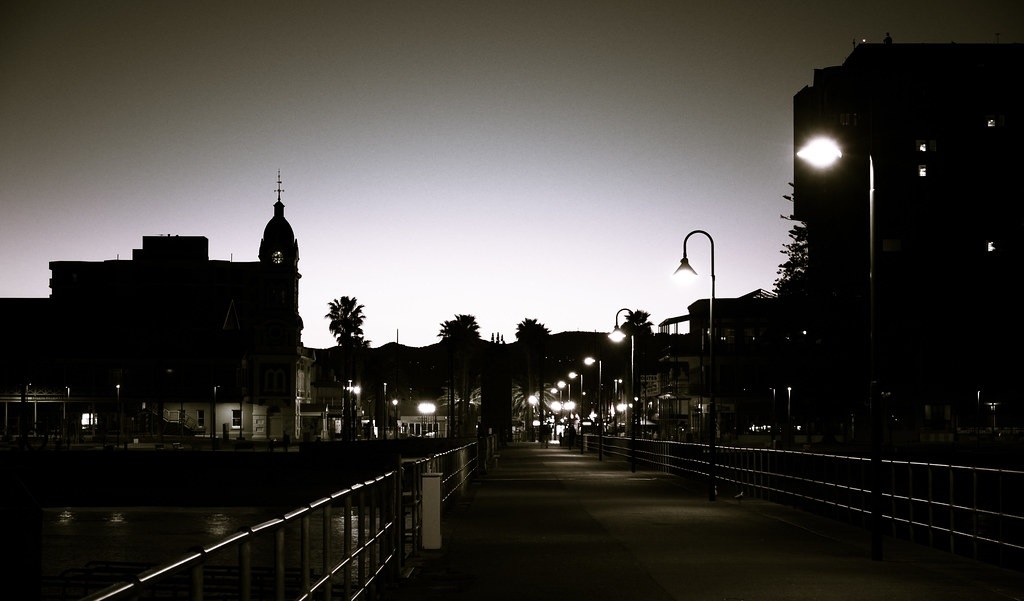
361;423;371;441
568;425;577;451
66;427;73;450
544;421;551;449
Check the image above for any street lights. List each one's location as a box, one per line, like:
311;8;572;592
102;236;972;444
391;398;399;439
795;121;884;560
786;386;792;447
567;366;584;455
550;382;564;447
112;382;122;447
557;376;572;450
608;308;637;474
583;352;603;462
769;386;777;419
614;377;623;438
211;383;221;450
669;230;717;503
